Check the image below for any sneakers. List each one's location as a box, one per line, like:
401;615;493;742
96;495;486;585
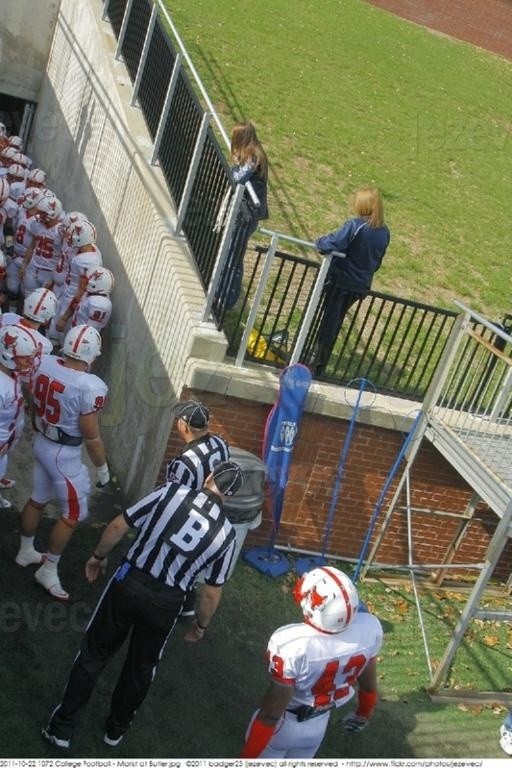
0;479;15;488
500;725;512;754
15;548;71;600
0;497;11;508
39;725;70;748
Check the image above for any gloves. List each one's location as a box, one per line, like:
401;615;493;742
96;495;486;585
338;711;369;734
95;461;111;488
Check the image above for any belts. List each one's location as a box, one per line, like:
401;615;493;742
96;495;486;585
286;704;333;723
33;412;82;446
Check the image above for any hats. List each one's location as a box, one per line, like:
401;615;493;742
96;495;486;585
214;461;244;496
172;399;210;427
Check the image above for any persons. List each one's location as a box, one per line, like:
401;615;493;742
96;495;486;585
211;123;268;311
1;118;114;601
165;401;230;618
304;188;391;373
241;566;384;758
41;461;245;747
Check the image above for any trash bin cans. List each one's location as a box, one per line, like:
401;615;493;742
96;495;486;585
223;446;269;581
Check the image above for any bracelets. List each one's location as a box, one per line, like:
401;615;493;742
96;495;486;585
197;621;207;630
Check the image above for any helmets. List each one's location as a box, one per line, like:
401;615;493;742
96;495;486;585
0;135;115;295
294;565;360;634
0;288;102;370
